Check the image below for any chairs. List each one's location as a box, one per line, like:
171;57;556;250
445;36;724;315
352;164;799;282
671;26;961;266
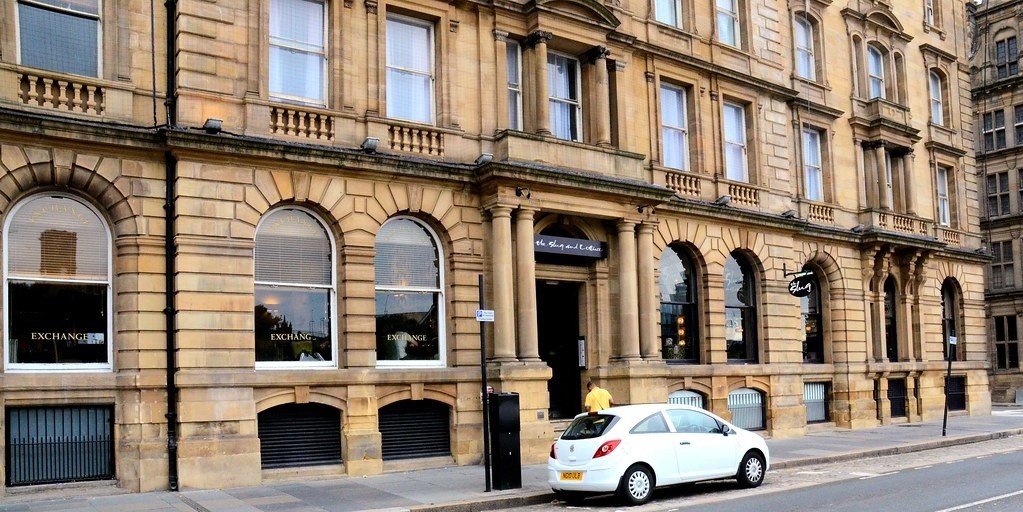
377;335;400;360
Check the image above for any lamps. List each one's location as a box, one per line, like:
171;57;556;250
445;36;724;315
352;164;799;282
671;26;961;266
203;118;224;134
360;137;380;154
975;246;987;254
851;224;864;233
716;195;731;207
806;324;812;331
475;153;493;166
782;208;798;219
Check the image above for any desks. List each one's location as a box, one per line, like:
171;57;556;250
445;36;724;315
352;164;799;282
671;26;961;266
406;345;438;360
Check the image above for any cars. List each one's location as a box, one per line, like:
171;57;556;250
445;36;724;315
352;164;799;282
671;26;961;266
546;403;770;505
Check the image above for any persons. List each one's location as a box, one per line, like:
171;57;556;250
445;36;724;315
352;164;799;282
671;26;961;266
254;305;275;361
394;328;418;360
583;381;614;412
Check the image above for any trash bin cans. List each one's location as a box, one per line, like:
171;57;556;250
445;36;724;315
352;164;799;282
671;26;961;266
488;392;522;490
